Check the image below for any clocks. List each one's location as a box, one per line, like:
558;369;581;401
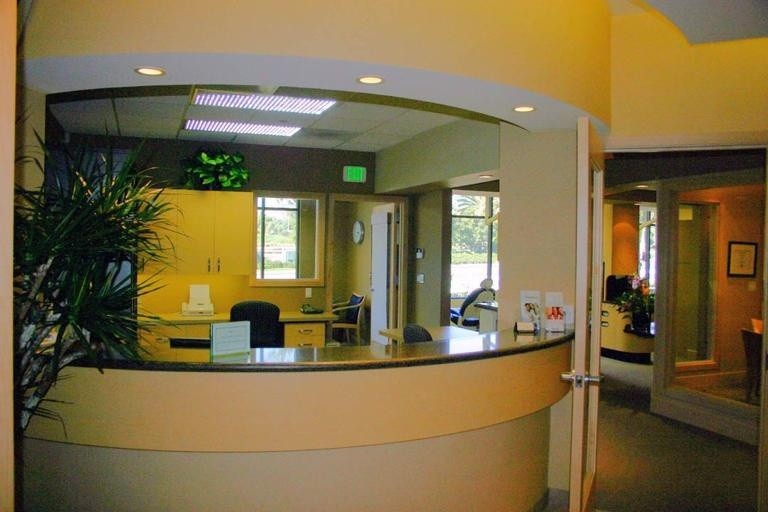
352;220;364;245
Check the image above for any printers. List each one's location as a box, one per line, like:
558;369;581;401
182;302;215;315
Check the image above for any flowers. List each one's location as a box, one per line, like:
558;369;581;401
615;274;654;314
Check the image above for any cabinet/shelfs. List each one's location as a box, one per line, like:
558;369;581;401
137;189;253;277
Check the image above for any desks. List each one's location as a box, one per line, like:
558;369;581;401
137;311;338;350
379;325;478;343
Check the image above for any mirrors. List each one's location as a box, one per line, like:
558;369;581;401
250;191;325;287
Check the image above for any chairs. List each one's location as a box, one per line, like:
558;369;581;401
403;326;432;342
740;328;767;401
450;278;496;331
231;301;280;349
333;293;365;347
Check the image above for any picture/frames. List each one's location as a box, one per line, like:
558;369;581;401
727;240;757;278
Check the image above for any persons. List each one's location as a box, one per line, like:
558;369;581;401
549;307;563;320
525;302;540;330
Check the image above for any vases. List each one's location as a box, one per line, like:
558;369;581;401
632;311;649;329
